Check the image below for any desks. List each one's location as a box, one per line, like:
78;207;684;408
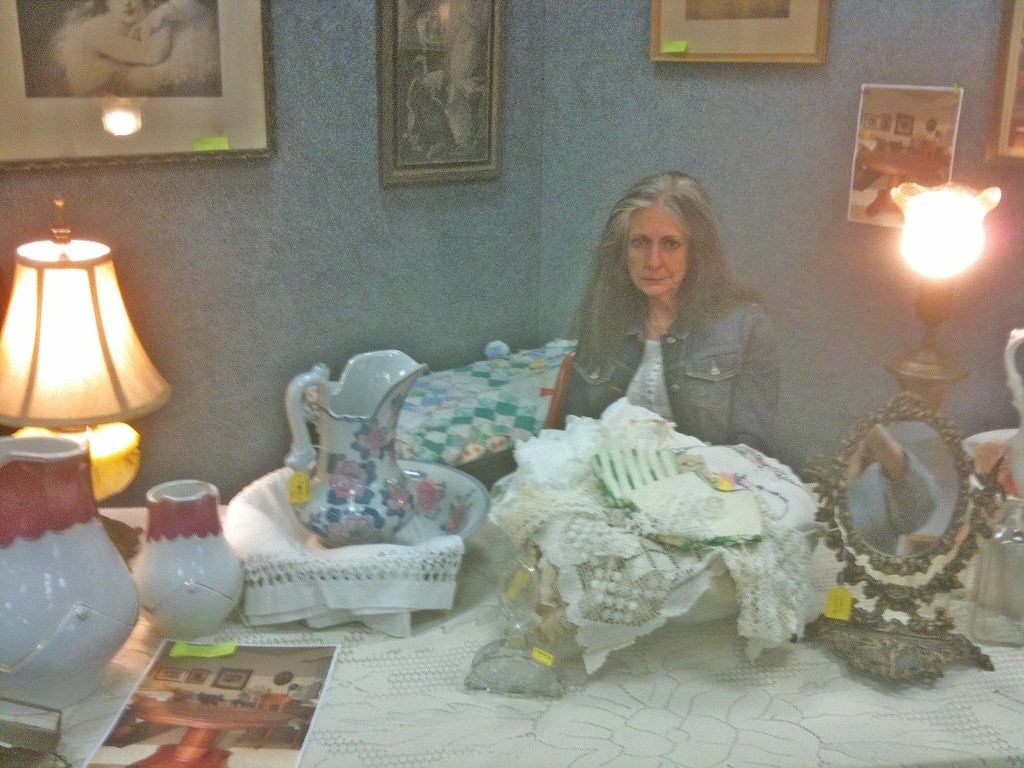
0;482;1024;768
395;338;580;493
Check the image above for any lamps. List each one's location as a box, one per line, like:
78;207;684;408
880;181;1001;417
0;195;174;561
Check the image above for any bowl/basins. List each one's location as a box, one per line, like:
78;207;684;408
396;458;493;541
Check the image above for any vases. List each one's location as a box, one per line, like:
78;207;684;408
0;436;138;709
133;478;245;640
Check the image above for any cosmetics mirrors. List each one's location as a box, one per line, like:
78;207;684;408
812;394;1006;689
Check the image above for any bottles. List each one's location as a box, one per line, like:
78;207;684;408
134;478;245;640
0;436;139;716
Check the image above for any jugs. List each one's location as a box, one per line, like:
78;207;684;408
282;349;428;549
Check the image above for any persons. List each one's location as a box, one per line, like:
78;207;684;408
563;171;780;460
848;423;939;556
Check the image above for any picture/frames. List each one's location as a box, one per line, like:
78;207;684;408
0;0;279;173
983;0;1024;168
375;0;507;191
650;0;831;65
